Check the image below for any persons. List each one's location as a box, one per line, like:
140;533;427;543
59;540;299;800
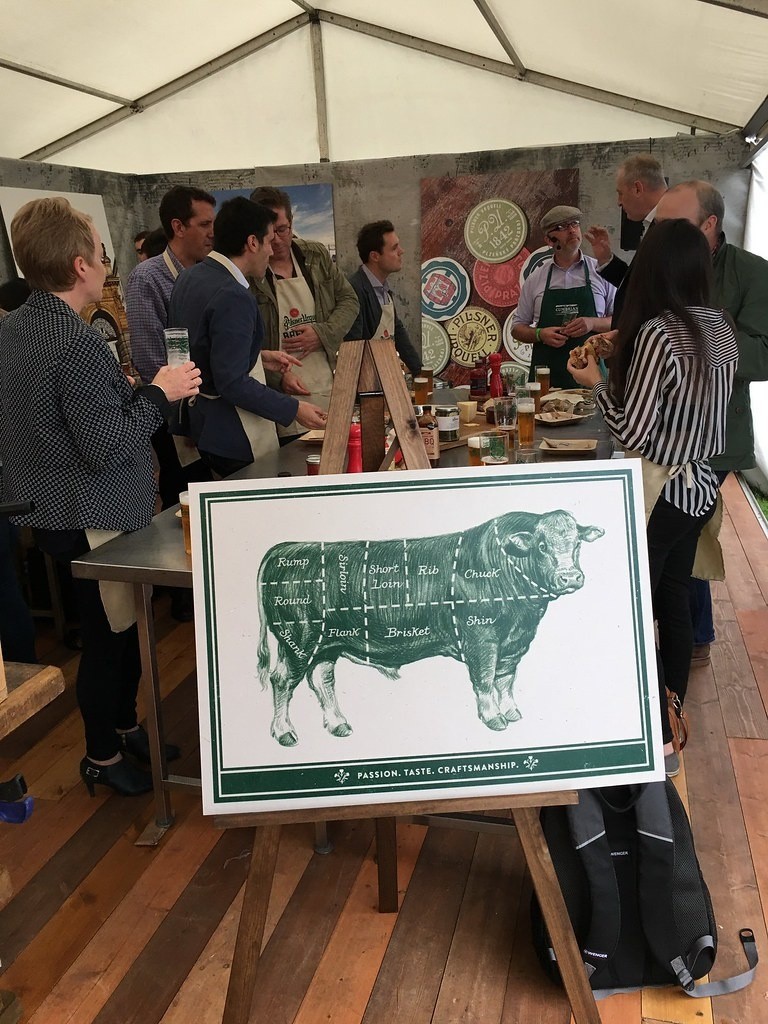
585;159;768;667
510;206;617;391
167;197;328;478
134;230;168;262
0;198;204;797
567;218;739;776
344;220;423;390
244;186;361;446
125;184;219;511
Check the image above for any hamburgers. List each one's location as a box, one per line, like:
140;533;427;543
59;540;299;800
569;338;614;369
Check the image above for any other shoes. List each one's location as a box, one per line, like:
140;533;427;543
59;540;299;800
665;751;681;779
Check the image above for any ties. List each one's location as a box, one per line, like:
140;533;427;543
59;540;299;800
383;289;389;305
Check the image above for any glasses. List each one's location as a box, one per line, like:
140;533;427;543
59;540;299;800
273;220;292;237
547;219;581;234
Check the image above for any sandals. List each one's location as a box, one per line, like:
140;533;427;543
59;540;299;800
690;642;711;666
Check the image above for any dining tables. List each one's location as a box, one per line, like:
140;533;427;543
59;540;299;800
68;394;647;836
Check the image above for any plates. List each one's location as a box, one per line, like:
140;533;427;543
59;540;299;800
534;412;586;426
175;509;183;518
538;438;598;454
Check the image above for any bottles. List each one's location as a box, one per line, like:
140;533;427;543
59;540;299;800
435;407;460;442
346;423;363;473
469;352;503;397
178;491;192;556
417;405;440;468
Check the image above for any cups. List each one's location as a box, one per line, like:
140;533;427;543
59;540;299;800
558;310;578;337
479;431;510;466
493;397;518;453
420;366;433;394
526;381;541;415
534;364;550;397
163;328;191;367
414;377;428;405
515;397;536;446
467;436;490;466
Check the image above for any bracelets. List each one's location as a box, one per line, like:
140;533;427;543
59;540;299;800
536;328;542;342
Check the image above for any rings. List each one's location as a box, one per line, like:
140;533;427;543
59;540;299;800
300;347;303;352
580;328;582;332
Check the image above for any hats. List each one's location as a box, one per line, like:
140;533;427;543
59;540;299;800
540;205;583;230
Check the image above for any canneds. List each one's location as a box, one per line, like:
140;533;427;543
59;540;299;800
305;454;322;475
434;405;461;443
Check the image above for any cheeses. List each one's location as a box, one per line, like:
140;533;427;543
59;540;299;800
457;401;478;423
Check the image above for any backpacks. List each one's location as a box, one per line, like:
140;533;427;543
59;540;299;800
525;777;759;1000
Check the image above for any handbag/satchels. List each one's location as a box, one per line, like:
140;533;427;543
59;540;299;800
663;685;691;830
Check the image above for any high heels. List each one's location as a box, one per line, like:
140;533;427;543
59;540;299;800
81;753;155;798
116;721;180;764
